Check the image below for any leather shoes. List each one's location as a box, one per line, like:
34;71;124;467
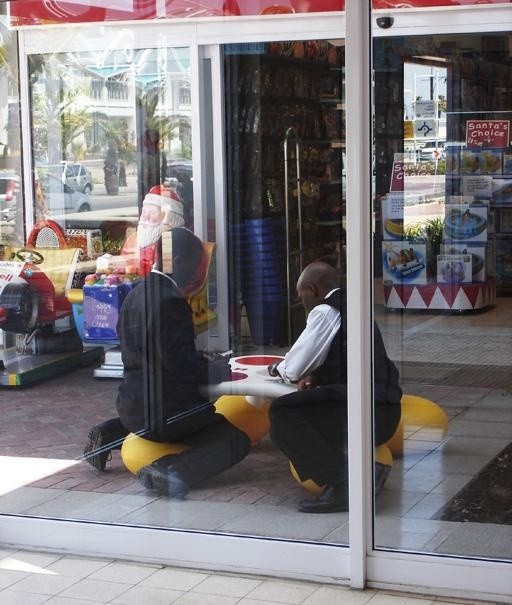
84;424;110;471
298;493;348;513
376;461;392;501
137;464;189;498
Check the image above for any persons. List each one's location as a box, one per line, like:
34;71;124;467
137;184;185;275
268;260;403;512
84;227;250;499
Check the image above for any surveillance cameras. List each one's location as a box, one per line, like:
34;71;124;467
376;17;394;29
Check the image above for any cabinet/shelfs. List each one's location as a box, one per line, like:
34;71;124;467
442;172;512;296
227;62;402;318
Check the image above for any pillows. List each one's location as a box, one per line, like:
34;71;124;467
3;245;84;300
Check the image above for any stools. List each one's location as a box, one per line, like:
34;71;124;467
290;394;449;492
120;429;194;473
213;395;272;449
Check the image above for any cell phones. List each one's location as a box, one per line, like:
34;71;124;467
219;349;234;357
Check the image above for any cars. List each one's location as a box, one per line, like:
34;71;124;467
0;163;94;216
159;156;192;180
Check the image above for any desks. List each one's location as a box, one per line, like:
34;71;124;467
197;354;300;411
